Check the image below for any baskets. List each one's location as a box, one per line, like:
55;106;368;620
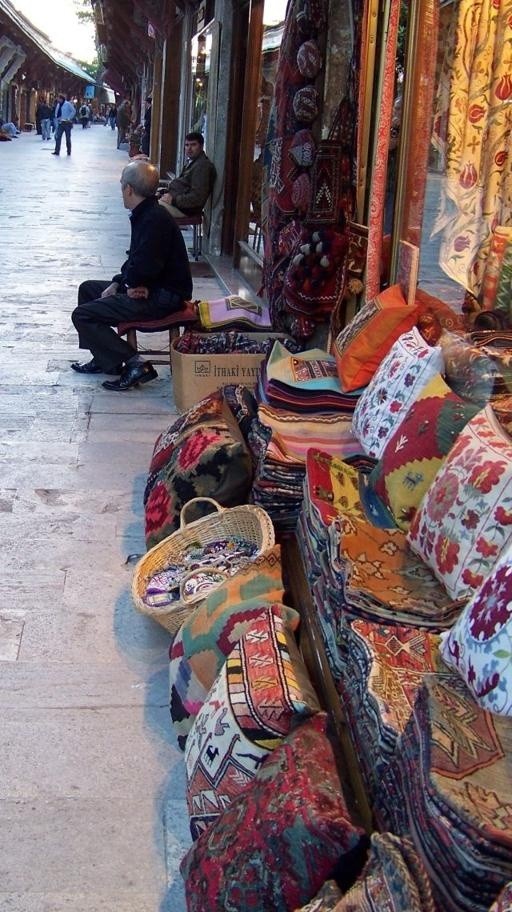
131;494;275;638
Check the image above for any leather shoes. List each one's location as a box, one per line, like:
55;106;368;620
71;355;124;377
102;361;159;391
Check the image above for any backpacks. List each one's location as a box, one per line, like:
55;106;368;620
81;105;88;116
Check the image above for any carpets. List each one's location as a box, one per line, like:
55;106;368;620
189;261;215;278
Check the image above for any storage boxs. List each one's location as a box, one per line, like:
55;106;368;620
172;332;300;413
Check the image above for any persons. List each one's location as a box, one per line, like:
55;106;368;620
72;160;191;389
12;118;21;133
1;122;18;138
127;133;217;256
35;94;151;160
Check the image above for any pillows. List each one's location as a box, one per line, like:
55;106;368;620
145;385;254;556
222;382;257;441
289;829;437;912
168;545;300;748
182;601;321;846
179;709;366;912
416;287;496;411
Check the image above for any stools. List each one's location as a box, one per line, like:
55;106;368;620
171;213;202;263
252;222;263;252
118;302;201;376
